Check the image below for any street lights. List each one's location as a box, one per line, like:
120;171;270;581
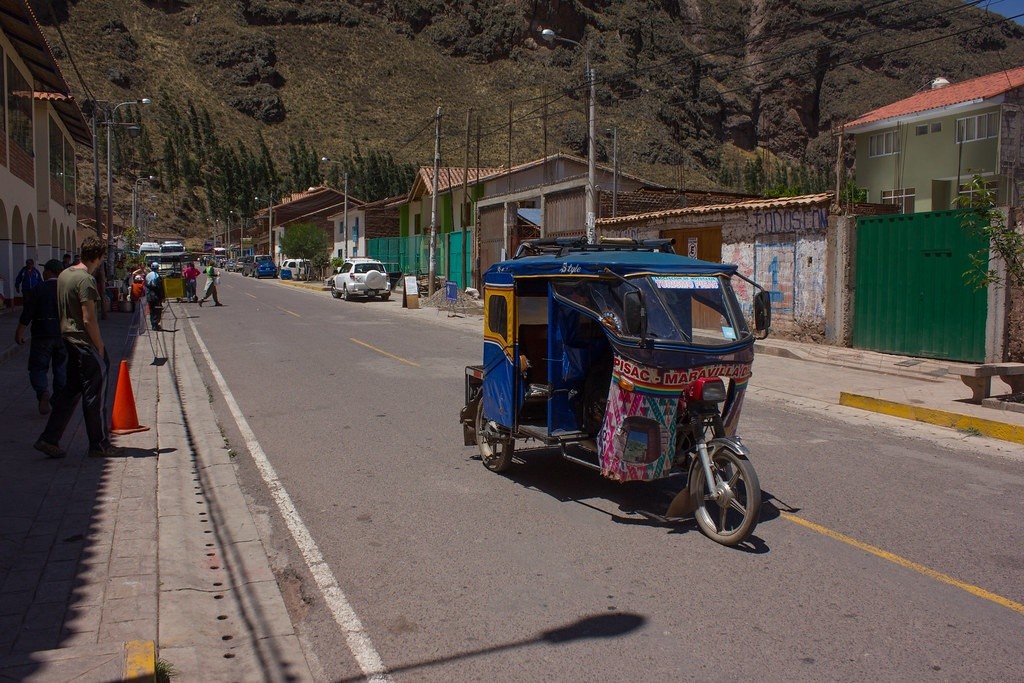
254;190;273;256
321;157;347;259
132;175;154;250
138;193;157;245
229;210;244;257
106;98;150;279
542;28;599;248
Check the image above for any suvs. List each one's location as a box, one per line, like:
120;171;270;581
330;257;402;302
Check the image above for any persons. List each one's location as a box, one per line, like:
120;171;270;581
115;261;164;331
197;260;223;307
196;256;224;269
33;234;128;458
14;254;81;415
184;261;201;303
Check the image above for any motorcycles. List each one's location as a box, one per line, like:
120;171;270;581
459;235;772;545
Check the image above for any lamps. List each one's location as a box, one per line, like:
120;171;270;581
67;203;73;215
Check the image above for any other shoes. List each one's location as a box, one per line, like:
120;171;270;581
152;326;162;331
33;438;66;458
198;300;203;307
37;394;53;415
88;446;126;458
215;303;222;306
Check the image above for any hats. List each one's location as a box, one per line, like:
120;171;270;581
134;275;144;281
38;259;64;275
150;262;160;269
73;255;81;260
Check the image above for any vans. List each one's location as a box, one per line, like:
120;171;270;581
279;258;314;282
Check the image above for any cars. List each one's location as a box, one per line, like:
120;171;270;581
255;261;278;279
135;240;273;277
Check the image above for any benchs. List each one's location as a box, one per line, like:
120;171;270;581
519;322;606;389
948;363;1024;404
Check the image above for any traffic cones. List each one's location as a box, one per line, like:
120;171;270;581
107;360;150;435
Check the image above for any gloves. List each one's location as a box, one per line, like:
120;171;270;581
15;324;27;346
